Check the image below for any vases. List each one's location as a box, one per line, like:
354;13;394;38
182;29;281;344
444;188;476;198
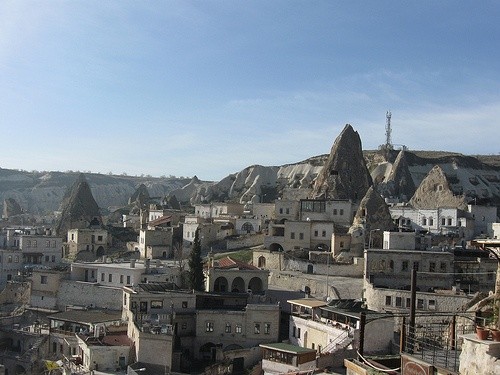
491;329;500;341
476;327;489;340
